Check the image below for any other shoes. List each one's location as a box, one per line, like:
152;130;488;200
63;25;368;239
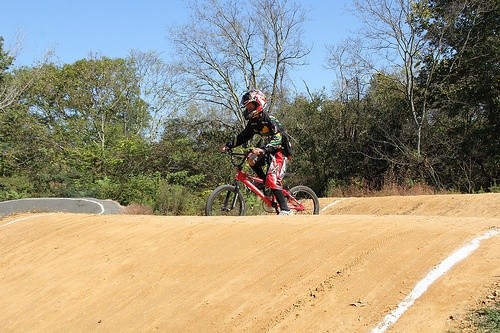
278;208;294;215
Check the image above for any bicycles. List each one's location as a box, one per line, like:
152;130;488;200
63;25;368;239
205;147;320;216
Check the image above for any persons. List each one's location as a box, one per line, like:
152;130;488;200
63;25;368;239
219;90;294;217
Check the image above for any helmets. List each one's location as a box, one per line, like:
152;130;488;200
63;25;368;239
240;89;268;120
